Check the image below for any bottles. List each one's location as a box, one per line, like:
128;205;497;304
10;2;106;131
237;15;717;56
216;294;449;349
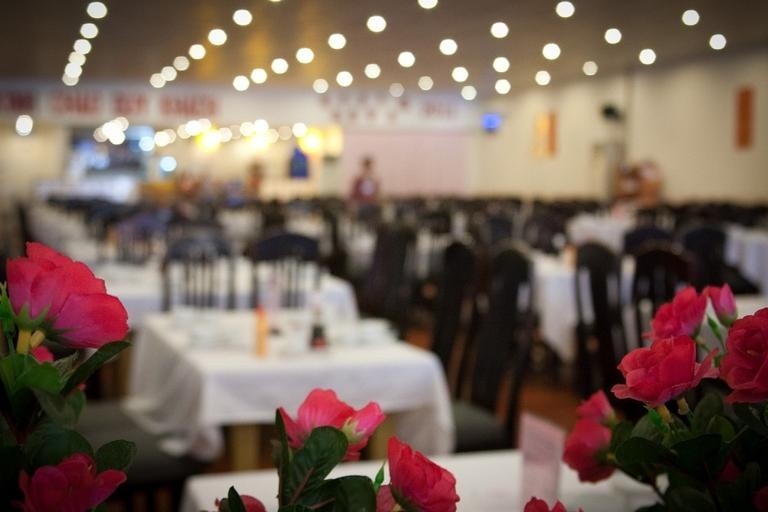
310;302;328;347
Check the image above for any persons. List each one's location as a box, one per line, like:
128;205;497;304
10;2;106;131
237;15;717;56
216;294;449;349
350;159;378;199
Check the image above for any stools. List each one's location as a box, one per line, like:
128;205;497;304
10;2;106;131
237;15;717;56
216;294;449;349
77;399;204;512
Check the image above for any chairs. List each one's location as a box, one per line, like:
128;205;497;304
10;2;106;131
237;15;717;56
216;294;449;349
44;184;768;456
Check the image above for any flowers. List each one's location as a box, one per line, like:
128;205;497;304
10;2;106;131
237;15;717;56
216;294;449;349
522;497;589;511
562;283;767;512
208;387;457;510
1;230;136;512
